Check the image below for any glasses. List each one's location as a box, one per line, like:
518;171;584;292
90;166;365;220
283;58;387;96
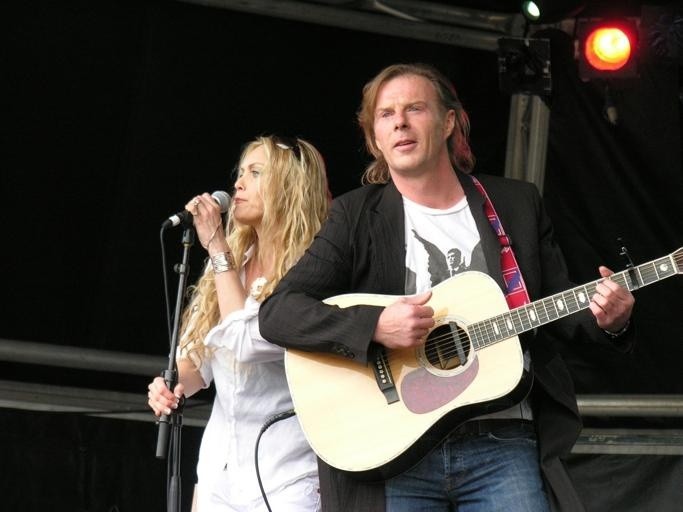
269;130;302;164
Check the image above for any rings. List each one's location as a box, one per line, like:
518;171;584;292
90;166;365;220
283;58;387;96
193;199;201;207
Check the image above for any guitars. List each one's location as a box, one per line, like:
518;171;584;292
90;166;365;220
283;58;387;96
284;245;683;483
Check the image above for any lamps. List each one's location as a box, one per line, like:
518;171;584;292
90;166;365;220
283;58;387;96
518;0;556;23
580;16;641;73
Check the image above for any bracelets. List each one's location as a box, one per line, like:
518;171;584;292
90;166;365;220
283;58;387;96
602;321;631;338
211;250;236;275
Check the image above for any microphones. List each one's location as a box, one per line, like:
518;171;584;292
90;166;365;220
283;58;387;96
173;189;233;225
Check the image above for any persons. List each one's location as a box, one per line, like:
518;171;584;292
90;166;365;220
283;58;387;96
255;63;636;511
145;134;334;512
446;246;468;276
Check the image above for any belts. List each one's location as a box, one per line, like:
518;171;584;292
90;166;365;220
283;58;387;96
451;414;538;438
